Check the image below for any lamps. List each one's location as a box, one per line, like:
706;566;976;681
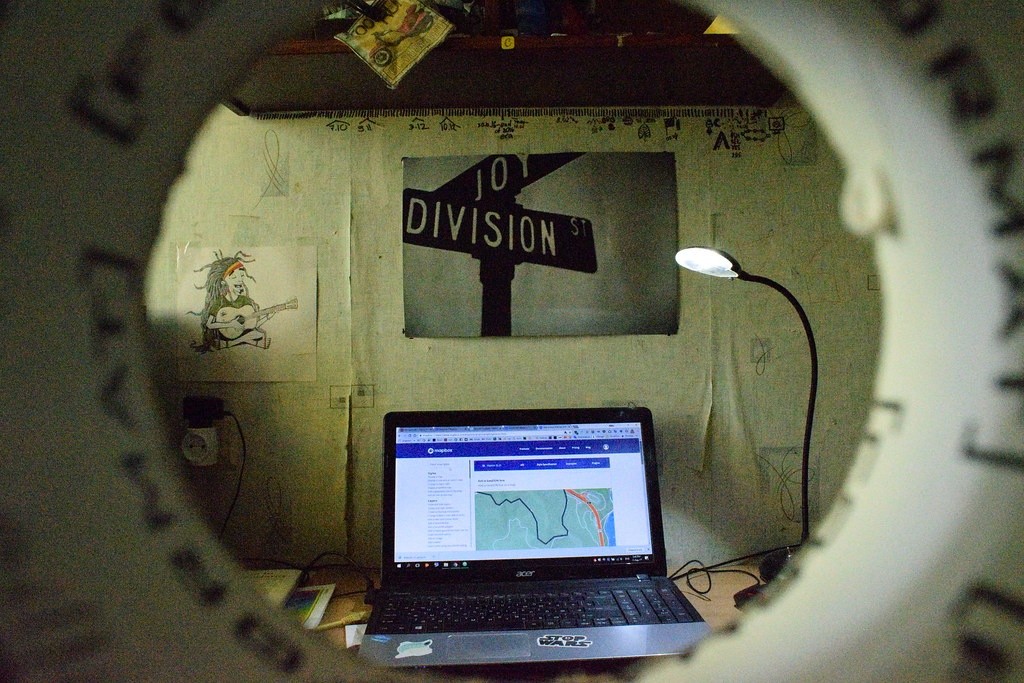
674;246;819;583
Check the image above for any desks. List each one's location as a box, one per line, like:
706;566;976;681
301;565;764;680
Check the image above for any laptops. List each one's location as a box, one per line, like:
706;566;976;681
356;407;714;666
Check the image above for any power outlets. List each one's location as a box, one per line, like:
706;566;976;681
189;397;241;473
